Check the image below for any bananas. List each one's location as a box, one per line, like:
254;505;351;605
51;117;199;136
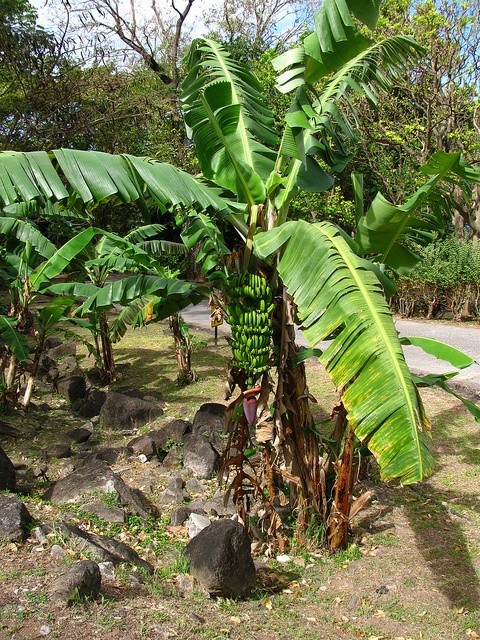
224;272;276;376
